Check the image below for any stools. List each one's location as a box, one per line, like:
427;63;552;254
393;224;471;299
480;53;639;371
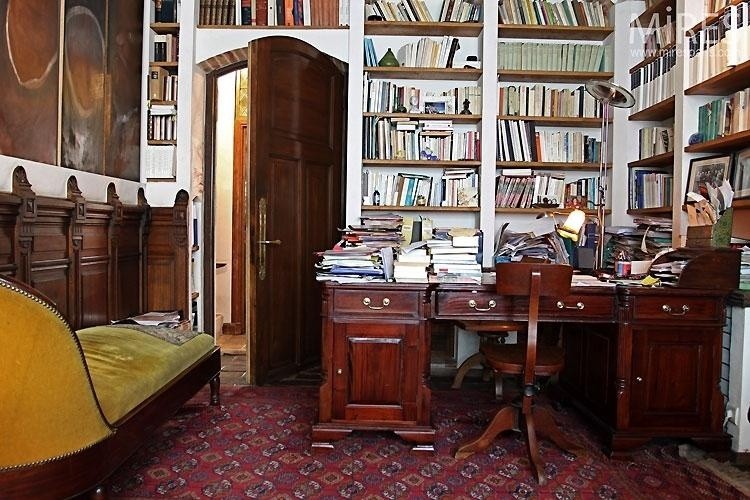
449;319;527;401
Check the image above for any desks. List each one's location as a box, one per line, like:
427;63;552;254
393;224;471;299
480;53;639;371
324;284;726;447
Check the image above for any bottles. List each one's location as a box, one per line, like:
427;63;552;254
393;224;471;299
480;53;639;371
374;190;380;206
417;195;426;206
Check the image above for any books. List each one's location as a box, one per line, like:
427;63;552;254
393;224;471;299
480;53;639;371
109;310;192;330
312;0;750;304
200;0;312;30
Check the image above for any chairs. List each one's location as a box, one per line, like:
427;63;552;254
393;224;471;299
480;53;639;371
457;258;588;486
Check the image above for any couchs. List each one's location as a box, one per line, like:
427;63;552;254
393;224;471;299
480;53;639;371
0;277;220;499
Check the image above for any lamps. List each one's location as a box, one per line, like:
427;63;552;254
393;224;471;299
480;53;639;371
583;82;637;278
558;208;604;284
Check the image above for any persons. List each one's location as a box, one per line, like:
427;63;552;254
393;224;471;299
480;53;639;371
148;2;180;144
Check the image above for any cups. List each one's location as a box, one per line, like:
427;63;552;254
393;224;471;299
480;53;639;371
615;262;630;277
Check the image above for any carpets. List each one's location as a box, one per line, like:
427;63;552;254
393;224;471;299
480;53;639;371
114;382;749;499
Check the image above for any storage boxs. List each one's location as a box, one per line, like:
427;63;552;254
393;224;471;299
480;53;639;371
674;244;743;292
685;225;713;251
729;199;750;246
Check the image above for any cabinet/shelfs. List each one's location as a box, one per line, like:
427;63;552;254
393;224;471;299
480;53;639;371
683;0;750;257
149;21;182;181
496;24;614;276
364;22;485;264
628;1;686;216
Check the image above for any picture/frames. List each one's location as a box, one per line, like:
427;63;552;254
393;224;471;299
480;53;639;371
733;149;750;199
684;153;733;203
